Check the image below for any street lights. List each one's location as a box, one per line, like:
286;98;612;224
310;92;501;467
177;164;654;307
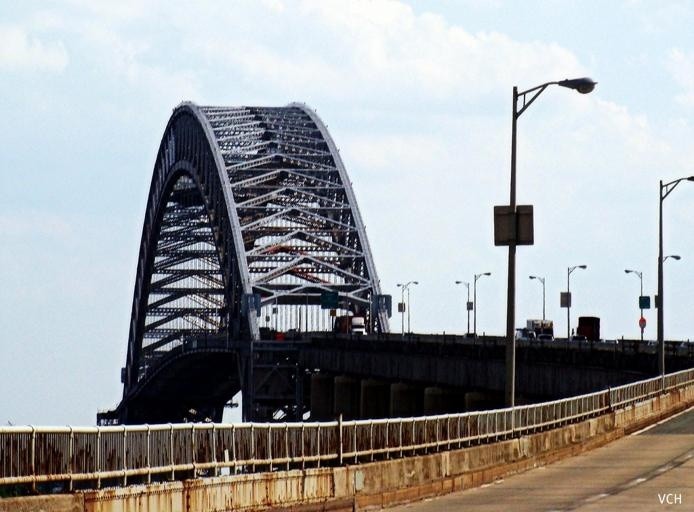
455;281;470;333
624;268;644;340
396;281;418;334
567;264;588;342
473;273;491;334
528;275;546;320
504;77;598;409
656;175;694;376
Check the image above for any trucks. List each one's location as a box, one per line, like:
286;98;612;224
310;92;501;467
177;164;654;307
333;315;367;336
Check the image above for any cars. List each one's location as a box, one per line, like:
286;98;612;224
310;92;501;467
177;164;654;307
516;316;688;348
